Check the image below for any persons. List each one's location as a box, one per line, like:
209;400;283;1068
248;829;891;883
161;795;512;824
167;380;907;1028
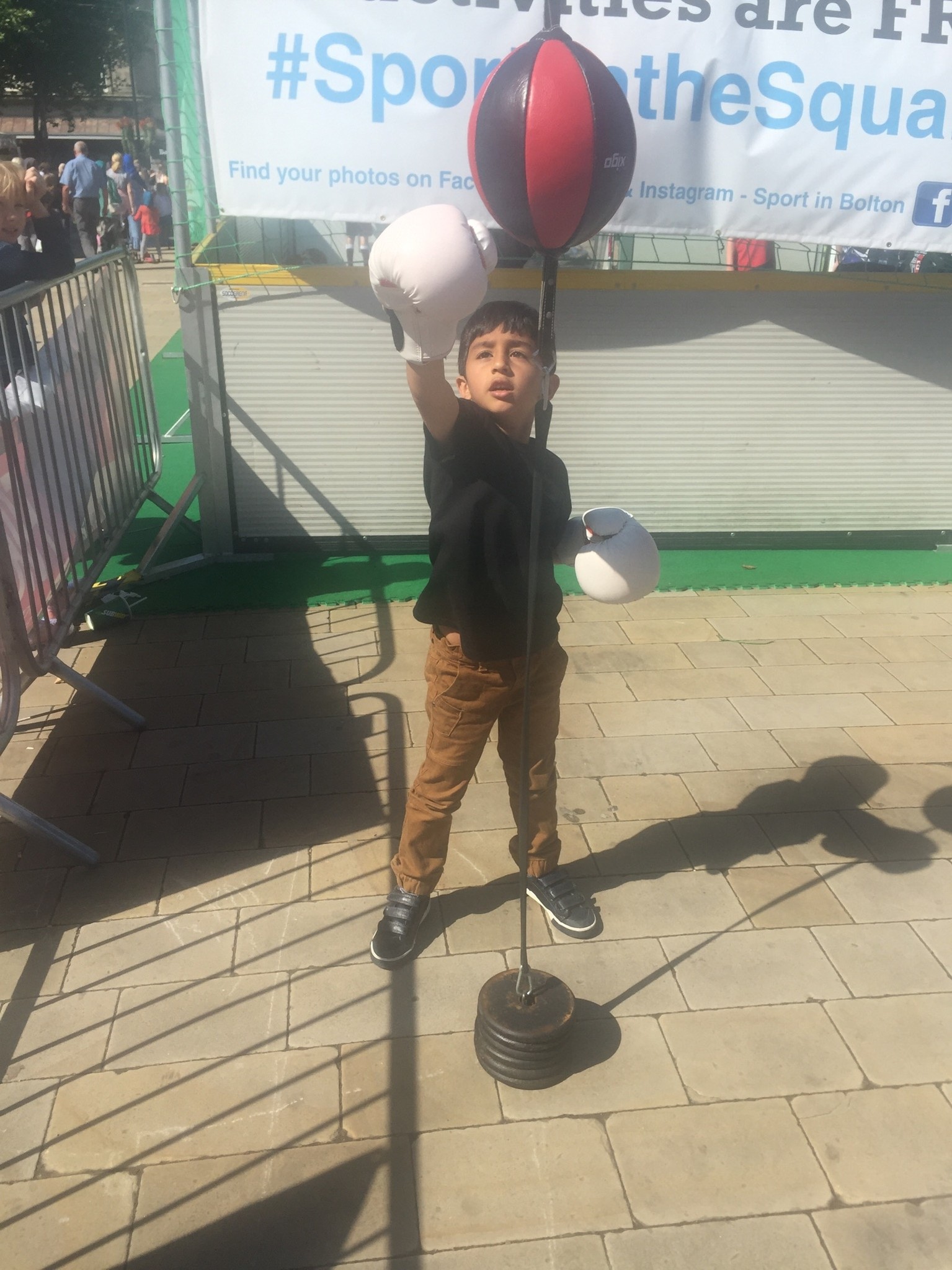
1;142;174;390
345;220;372;267
724;237;778;270
369;206;658;970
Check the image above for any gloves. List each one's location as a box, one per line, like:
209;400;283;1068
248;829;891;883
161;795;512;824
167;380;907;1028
369;205;499;366
557;506;658;605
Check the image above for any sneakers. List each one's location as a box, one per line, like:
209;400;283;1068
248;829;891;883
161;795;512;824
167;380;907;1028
370;884;433;968
526;866;605;936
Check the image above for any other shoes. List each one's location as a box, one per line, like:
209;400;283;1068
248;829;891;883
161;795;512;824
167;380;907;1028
25;619;75;645
